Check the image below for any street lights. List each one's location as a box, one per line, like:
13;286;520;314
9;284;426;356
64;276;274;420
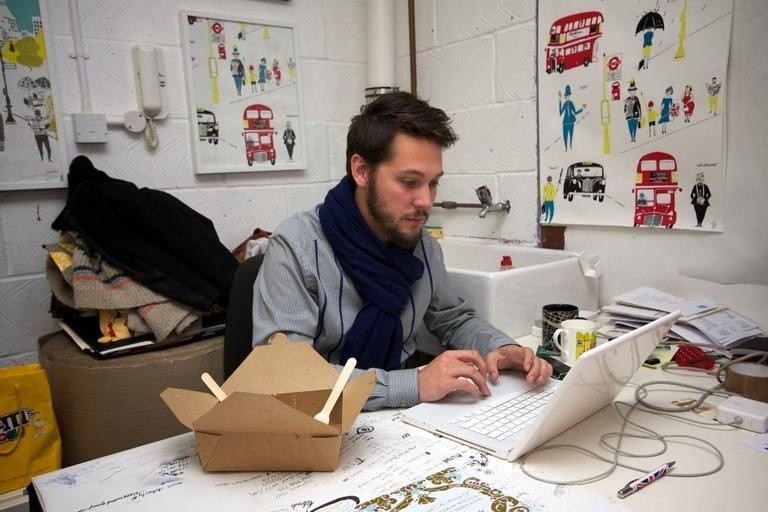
1;364;63;494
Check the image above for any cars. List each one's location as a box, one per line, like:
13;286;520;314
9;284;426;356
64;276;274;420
539;302;589;352
552;320;599;365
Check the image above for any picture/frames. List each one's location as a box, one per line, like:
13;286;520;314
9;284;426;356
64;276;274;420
28;305;766;511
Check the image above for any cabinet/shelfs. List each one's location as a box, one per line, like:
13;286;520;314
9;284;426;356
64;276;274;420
499;255;515;271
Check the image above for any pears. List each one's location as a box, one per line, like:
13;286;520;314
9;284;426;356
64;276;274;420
497;254;516;272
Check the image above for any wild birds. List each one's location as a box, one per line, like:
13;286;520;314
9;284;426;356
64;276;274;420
400;309;682;462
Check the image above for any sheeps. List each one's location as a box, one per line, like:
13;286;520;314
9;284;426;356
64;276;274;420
475;184;510;219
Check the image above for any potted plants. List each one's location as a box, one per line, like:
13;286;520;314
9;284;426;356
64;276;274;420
0;1;71;191
177;9;308;175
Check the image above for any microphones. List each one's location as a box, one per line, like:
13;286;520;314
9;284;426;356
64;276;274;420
34;328;223;468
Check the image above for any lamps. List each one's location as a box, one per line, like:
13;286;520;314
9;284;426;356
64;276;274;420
222;251;265;378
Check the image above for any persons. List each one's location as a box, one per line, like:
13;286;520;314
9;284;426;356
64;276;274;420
252;92;553;412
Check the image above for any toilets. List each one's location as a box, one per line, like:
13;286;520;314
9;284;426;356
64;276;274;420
131;44;170;120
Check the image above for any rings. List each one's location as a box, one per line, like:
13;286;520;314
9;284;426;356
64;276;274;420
617;461;676;499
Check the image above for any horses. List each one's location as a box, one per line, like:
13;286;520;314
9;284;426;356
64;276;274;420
539;354;570;380
